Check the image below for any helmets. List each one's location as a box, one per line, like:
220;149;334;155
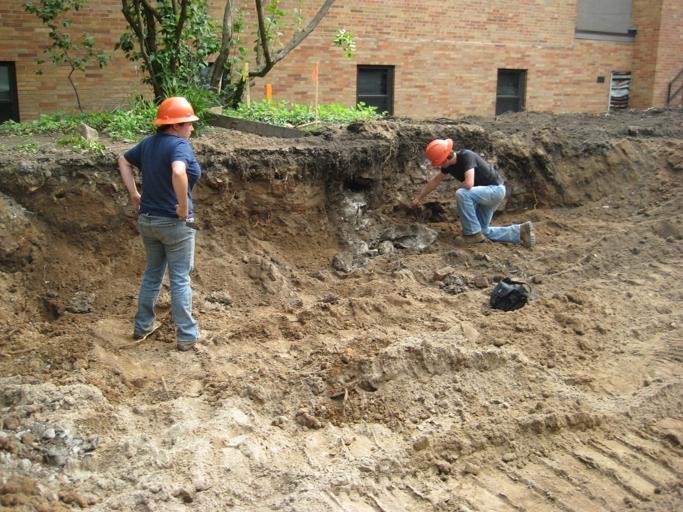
152;96;200;125
426;138;454;168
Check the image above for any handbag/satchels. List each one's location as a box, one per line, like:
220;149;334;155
490;277;532;313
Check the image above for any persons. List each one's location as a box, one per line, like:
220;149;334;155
408;139;536;249
117;96;201;352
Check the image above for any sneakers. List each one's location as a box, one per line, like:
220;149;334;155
519;221;535;250
462;231;485;244
133;320;162;340
